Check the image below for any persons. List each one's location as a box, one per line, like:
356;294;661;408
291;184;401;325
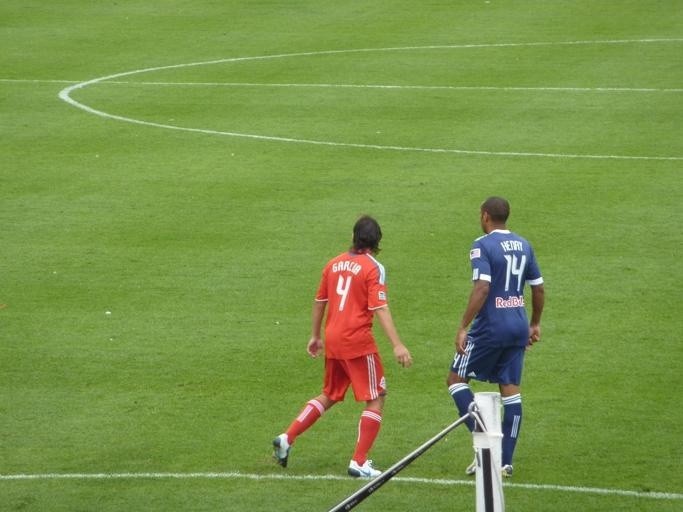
272;215;412;478
446;196;545;479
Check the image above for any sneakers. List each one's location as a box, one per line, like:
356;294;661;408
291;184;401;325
500;464;513;477
347;458;381;477
272;433;291;467
465;459;477;474
